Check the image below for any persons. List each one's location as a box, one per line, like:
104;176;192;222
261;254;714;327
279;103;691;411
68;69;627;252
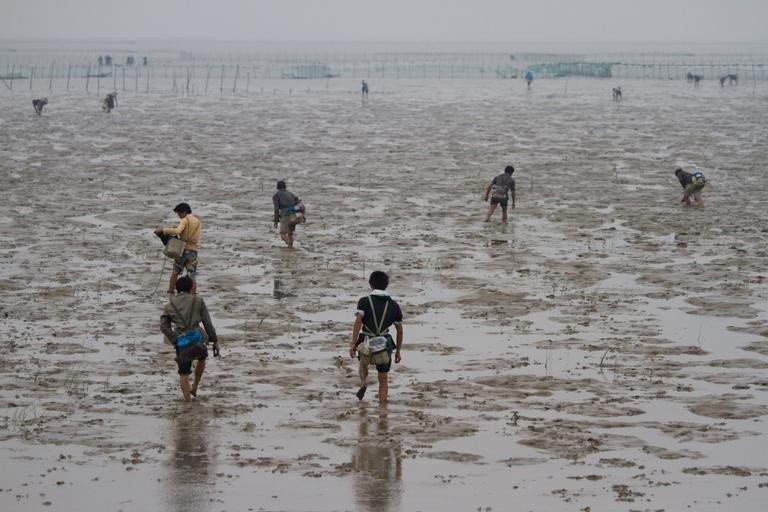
482;164;516;225
719;75;727;86
272;180;306;250
693;74;700;88
101;92;119;114
686;72;691;83
350;406;402;512
729;74;737;86
31;97;48;116
611;87;622;102
160;277;221;406
525;72;533;91
153;200;202;296
674;166;707;209
360;79;370;100
348;269;404;410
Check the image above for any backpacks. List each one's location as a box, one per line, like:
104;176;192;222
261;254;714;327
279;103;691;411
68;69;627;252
176;329;208;360
287;204;305;223
491;184;508;200
